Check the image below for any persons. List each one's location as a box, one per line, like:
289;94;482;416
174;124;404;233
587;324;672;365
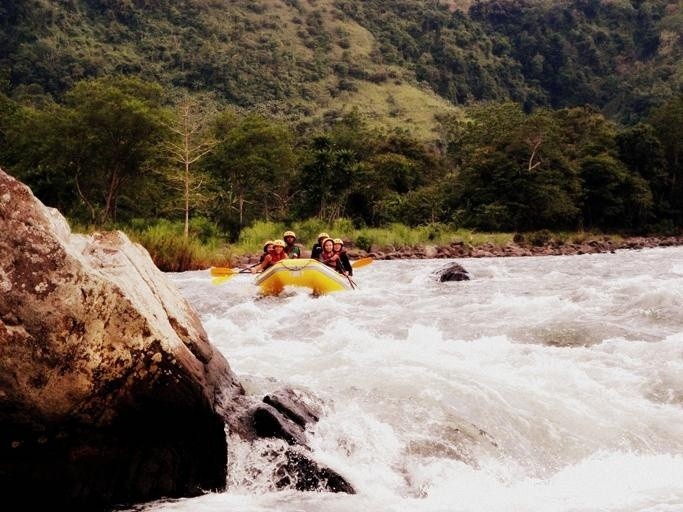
247;229;352;281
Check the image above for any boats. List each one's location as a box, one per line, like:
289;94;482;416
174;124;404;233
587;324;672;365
251;256;355;299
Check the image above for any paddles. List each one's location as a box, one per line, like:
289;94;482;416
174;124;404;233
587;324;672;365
211;262;262;286
350;256;372;268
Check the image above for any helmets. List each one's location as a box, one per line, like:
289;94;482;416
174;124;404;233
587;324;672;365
319;232;344;250
284;231;296;239
273;239;285;249
264;240;273;247
